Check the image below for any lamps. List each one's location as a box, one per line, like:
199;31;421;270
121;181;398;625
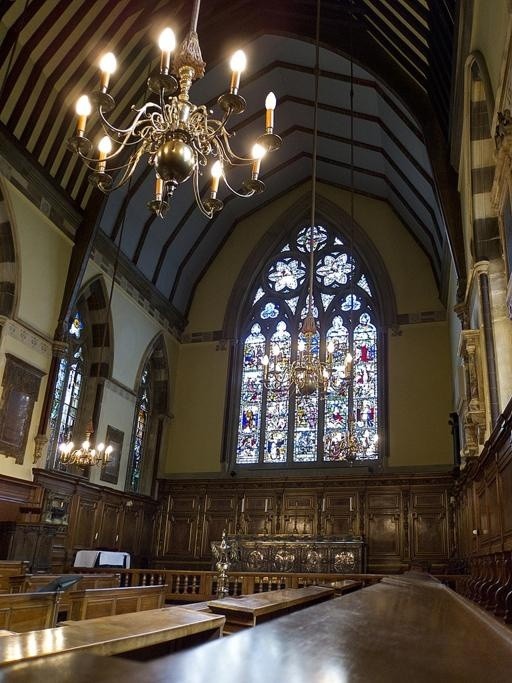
72;0;359;401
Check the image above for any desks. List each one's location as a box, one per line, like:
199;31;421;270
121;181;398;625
1;607;226;671
1;561;169;632
207;579;363;637
150;570;512;682
